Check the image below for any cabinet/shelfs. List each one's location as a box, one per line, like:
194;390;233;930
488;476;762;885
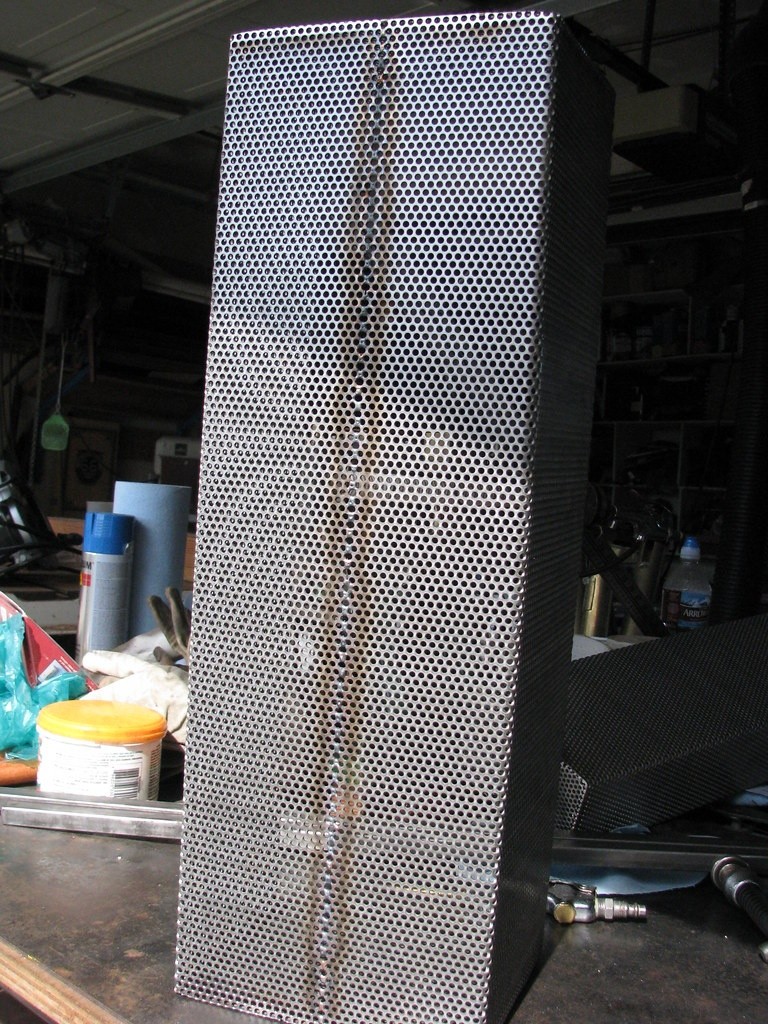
595;281;744;535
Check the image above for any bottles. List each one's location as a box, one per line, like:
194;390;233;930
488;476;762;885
658;538;714;632
